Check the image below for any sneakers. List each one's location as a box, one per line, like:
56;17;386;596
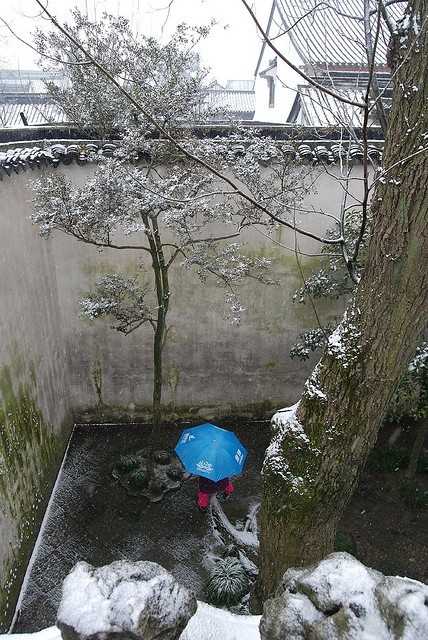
223;492;230;499
200;505;207;512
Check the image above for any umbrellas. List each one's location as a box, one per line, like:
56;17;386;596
173;422;248;483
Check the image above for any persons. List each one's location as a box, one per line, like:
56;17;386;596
198;478;234;512
